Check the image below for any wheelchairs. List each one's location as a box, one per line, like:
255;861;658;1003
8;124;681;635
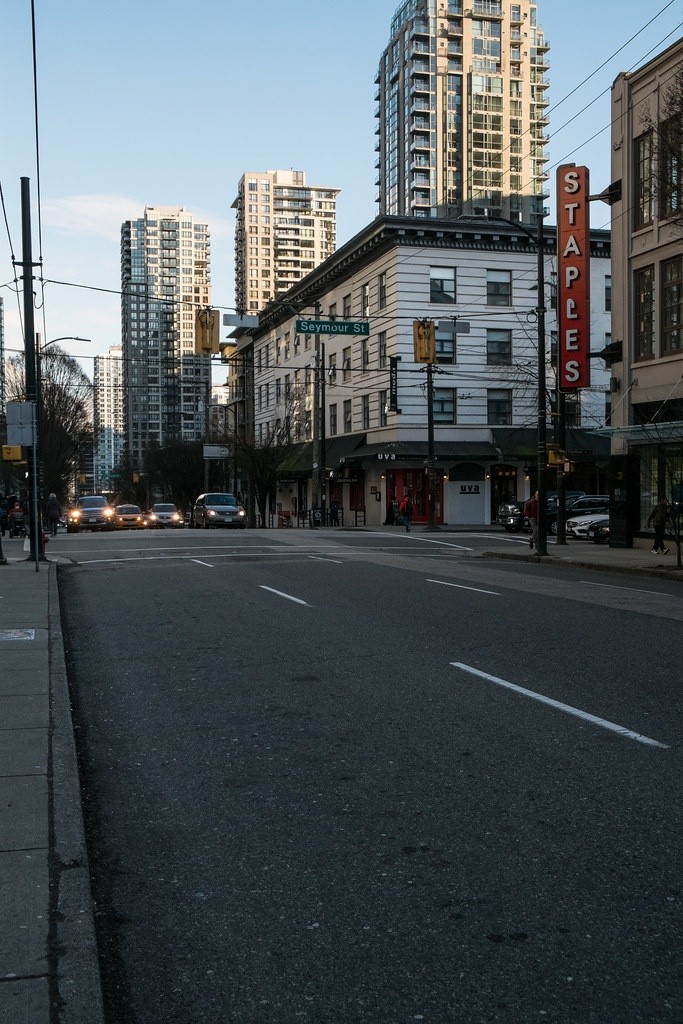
7;512;27;537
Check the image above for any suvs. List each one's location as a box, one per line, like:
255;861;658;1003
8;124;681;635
495;487;585;535
65;495;114;533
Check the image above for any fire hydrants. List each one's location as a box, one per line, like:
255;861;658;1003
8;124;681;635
41;528;50;561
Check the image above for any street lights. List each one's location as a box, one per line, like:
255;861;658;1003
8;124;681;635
456;209;552;557
34;331;92;514
204;402;238;495
526;281;567;544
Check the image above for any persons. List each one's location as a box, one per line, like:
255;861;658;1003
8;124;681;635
399;495;413;532
0;491;30;535
330;495;340;527
46;493;62;537
525;491;539;548
647;496;671;556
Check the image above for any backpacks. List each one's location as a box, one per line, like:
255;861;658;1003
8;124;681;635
405;500;413;515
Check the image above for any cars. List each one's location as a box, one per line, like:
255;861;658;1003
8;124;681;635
112;504;148;529
546;495;610;543
147;503;184;528
192;492;248;529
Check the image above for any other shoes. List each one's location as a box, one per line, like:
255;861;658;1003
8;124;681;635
651;549;658;554
663;548;670;555
529;539;533;548
